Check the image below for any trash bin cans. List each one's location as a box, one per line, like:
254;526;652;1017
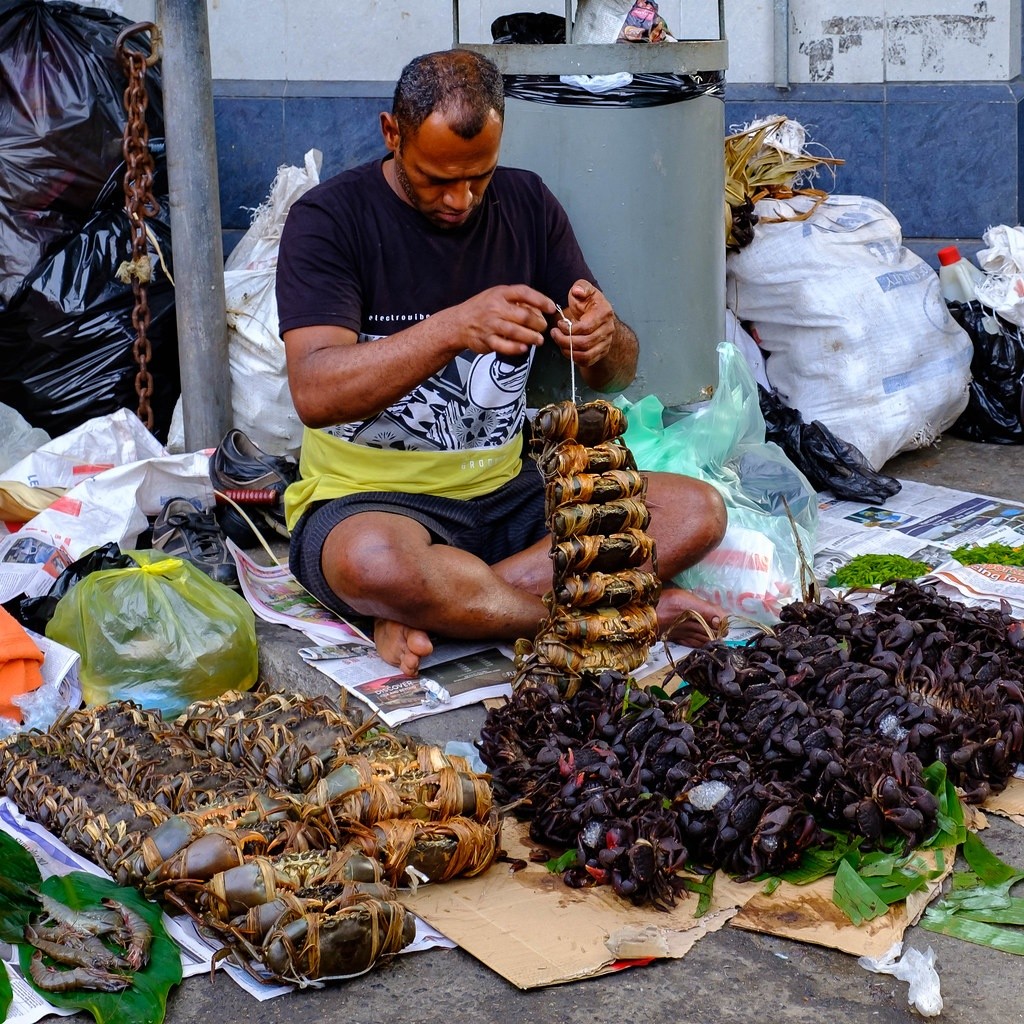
451;0;729;407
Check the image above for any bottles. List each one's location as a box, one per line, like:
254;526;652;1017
937;246;986;302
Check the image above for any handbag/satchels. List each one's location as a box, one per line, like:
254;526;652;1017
611;339;818;627
43;543;258;719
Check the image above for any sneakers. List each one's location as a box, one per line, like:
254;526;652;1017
150;496;240;588
209;428;301;538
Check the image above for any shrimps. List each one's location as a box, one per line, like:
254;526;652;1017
28;889;116;935
23;925;103;970
29;949;133;992
100;896;153;971
79;910;133;947
30;913;91;950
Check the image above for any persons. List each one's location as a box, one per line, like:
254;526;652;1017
275;49;728;679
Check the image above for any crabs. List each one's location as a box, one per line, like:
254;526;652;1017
0;397;1024;988
